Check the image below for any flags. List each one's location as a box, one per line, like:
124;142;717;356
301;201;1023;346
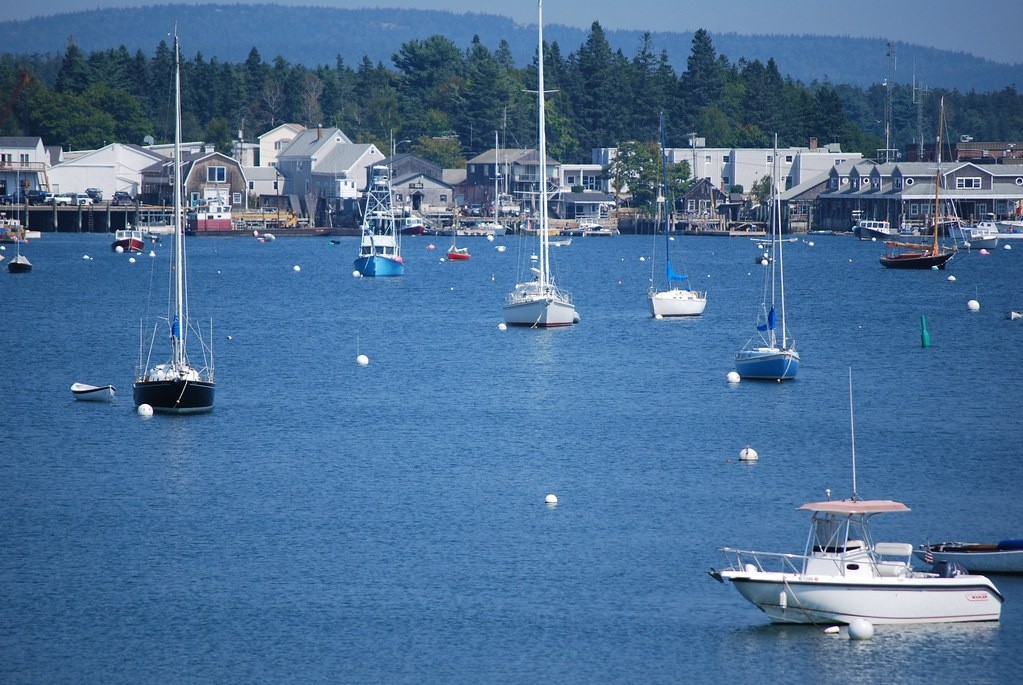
924;542;934;563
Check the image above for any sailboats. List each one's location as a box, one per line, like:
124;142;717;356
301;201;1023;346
921;133;967;235
446;197;472;260
133;33;218;415
877;94;970;269
728;132;799;383
8;143;33;274
650;109;710;318
478;130;508;237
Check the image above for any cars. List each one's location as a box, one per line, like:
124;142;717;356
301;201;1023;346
459;203;494;215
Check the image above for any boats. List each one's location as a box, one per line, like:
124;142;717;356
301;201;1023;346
854;219;897;241
111;229;145;254
808;230;834;235
398;216;427;236
71;380;115;404
949;222;1023;249
356;165;404;277
0;212;26;243
915;540;1023;576
186;198;336;237
709;497;1004;626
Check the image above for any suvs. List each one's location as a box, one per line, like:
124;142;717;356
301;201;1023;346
0;187;133;206
